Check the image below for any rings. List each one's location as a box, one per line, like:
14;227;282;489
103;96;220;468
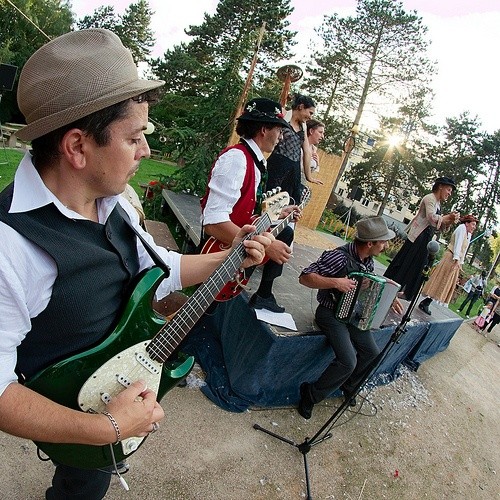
151;422;159;433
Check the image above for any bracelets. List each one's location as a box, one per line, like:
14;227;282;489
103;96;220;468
101;411;120;445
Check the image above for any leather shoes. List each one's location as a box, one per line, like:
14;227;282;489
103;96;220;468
249;290;286;313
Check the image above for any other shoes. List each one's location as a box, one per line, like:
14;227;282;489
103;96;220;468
343;386;358;406
419;299;432;314
466;316;468;318
478;330;482;335
456;309;461;312
471;325;475;329
300;382;312;421
484;335;487;337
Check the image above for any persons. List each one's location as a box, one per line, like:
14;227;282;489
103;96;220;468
250;97;324;312
288;119;325;254
299;216;403;419
457;270;488;318
383;177;460;301
419;215;479;315
471;285;500;337
0;28;276;500
177;98;303;388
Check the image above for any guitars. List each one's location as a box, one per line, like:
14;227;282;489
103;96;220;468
200;185;311;303
20;184;291;471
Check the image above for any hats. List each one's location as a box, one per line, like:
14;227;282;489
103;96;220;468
355;215;396;240
236;98;290;129
460;215;479;224
434;176;457;191
15;27;167;141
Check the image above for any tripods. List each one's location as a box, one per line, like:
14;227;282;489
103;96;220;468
252;261;433;499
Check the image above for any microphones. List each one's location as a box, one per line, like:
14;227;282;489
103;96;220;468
424;239;440;280
